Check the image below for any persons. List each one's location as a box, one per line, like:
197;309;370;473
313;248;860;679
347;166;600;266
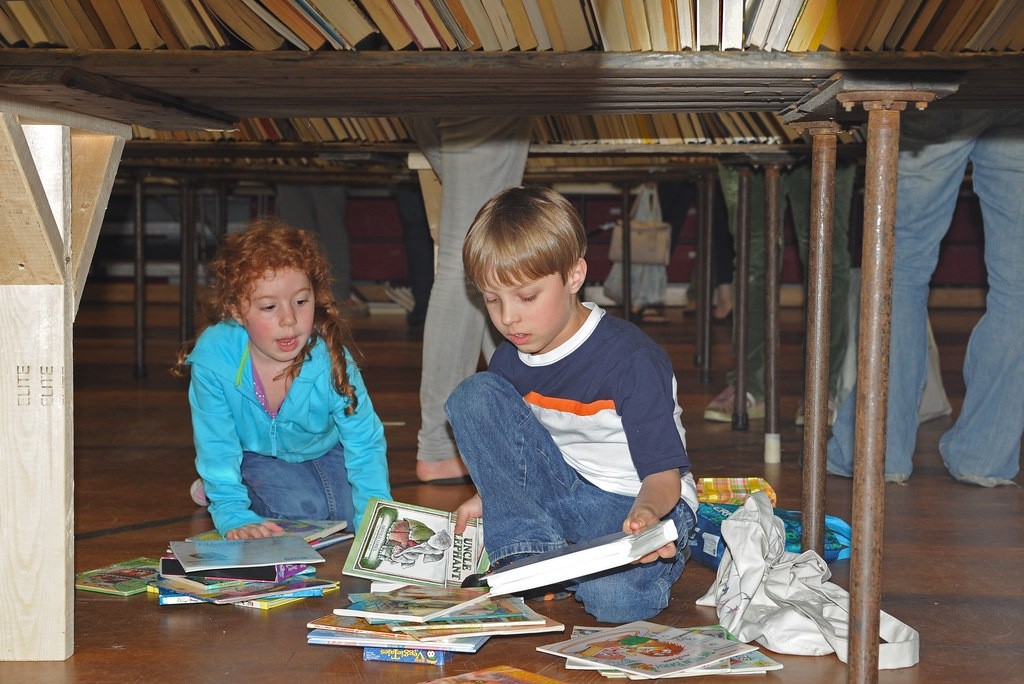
441;182;705;627
185;216;400;545
263;108;1024;480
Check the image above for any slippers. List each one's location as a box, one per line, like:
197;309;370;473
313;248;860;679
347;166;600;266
387;466;475;489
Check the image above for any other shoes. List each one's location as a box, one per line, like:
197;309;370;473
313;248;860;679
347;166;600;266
683;301;733;326
628;302;669;322
190;477;209;506
336;299;371;321
407;305;432;340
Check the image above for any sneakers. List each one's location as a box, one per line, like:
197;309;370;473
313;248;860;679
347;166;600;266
797;389;836;426
704;385;778;421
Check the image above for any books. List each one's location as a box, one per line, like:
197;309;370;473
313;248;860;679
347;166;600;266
74;496;786;682
